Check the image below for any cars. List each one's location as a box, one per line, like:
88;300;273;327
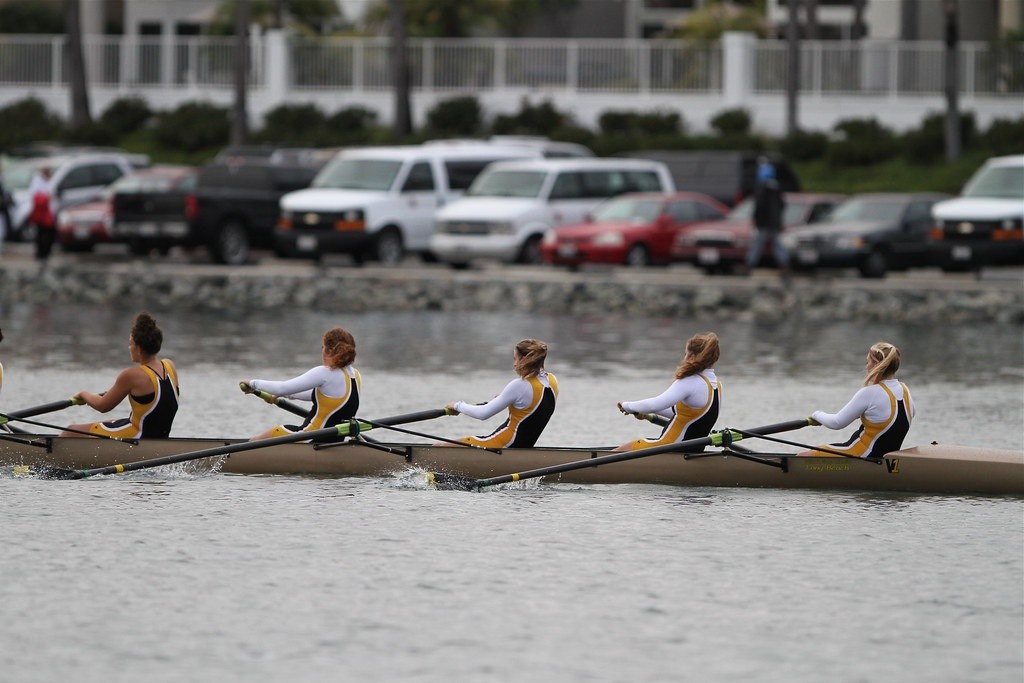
772;191;945;279
675;193;850;276
540;191;759;271
929;155;1024;273
5;151;131;243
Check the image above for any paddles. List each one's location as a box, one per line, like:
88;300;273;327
241;382;310;417
15;402;494;481
618;403;756;454
0;391;108;425
425;413;859;489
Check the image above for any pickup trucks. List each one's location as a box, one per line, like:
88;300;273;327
108;145;333;264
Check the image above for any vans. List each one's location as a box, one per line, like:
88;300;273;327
271;135;594;266
431;157;679;266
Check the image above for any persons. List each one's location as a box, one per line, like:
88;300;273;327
609;332;722;452
28;166;54;262
59;313;179;438
238;329;361;443
746;177;788;266
798;342;915;457
433;339;560;449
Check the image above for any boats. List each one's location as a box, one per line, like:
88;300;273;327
15;433;1023;498
